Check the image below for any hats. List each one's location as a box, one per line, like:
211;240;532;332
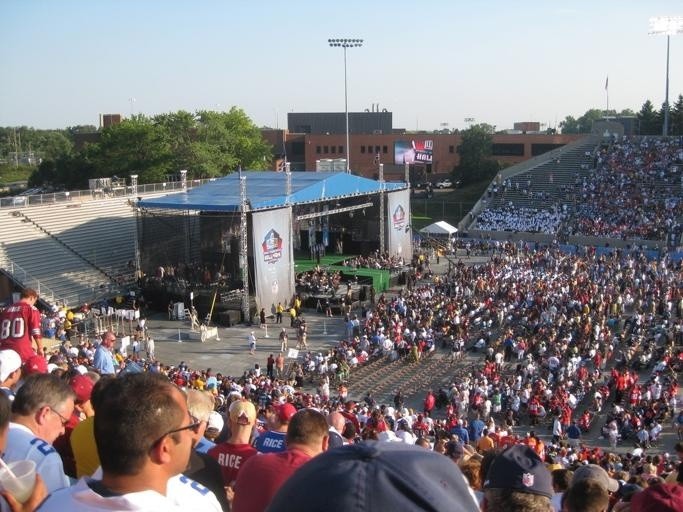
484;443;553;497
229;400;256;426
265;439;482;512
570;464;619;493
631;483;682;510
272;402;297;420
0;351;98;407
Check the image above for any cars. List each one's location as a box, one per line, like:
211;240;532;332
416;181;433;188
435;178;460;190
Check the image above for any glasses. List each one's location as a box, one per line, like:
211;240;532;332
49;408;71;426
162;417;200;439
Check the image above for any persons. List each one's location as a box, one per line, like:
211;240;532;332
1;197;399;511
398;131;682;511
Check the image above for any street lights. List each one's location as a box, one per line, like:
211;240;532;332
647;14;682;134
327;37;365;170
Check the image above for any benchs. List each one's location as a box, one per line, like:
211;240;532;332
484;143;682;208
341;351;483;398
0;188;200;307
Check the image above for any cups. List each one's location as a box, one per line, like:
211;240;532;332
0;461;37;503
